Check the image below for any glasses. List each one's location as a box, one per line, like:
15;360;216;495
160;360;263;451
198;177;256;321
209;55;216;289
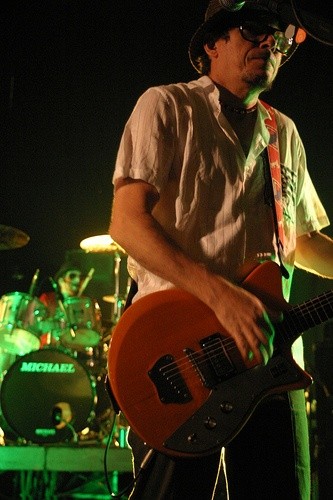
228;19;292;53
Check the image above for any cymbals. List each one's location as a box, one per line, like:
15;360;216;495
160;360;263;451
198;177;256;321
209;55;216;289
0;224;29;249
80;234;119;252
102;295;126;304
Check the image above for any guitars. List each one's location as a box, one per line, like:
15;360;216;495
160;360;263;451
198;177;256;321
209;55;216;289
106;260;333;457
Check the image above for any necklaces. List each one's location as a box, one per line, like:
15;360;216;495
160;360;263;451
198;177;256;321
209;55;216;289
223;101;259;114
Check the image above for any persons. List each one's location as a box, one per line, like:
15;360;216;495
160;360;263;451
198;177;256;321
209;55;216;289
108;0;333;500
26;259;105;339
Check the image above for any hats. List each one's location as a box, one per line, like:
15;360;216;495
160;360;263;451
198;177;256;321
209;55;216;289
188;0;299;75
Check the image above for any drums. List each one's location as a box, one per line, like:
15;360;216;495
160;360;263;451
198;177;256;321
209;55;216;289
55;296;103;349
0;347;98;443
0;292;50;356
